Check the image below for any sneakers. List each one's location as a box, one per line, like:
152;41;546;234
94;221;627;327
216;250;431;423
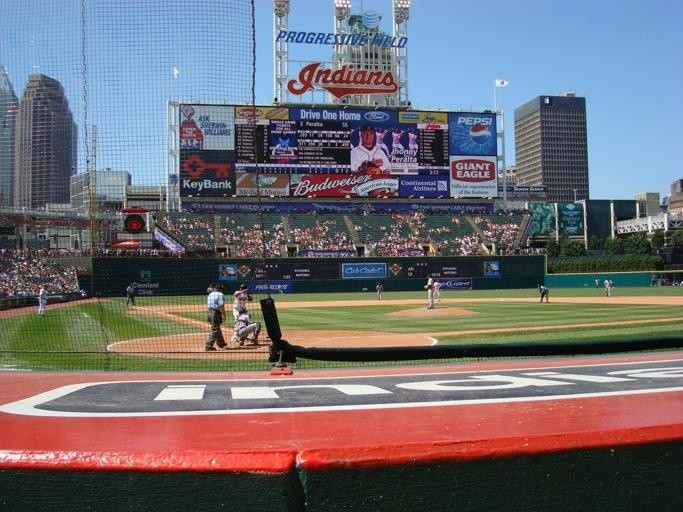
204;343;227;351
232;336;258;345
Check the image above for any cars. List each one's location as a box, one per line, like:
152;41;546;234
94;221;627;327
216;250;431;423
664;280;671;285
672;280;680;286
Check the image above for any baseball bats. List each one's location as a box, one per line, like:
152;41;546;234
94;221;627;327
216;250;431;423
249;268;258;288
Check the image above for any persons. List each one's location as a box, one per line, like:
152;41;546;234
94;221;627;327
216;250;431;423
432;278;441;302
375;282;384;300
230;283;253;320
36;284;49;317
603;277;612;297
350;124;390;174
219;265;236;280
162;209;542;259
388;127;407;157
407;128;418;150
0;244;158;297
537;283;550;303
423;274;434;310
230;306;261;345
203;283;227;351
125;281;136;306
594;277;599;288
374;127;392;159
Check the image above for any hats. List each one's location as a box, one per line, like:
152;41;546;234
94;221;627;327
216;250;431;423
360;124;375;132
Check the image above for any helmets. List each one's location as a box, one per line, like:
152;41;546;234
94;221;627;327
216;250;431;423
239;307;246;315
240;284;248;291
